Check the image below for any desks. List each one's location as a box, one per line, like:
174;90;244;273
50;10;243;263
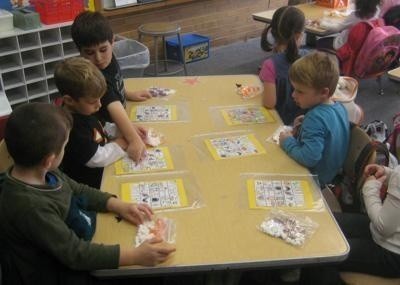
252;0;381;50
90;74;351;283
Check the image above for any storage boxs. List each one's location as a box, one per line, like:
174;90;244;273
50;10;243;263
31;0;85;25
11;8;41;28
0;9;14;31
165;32;210;65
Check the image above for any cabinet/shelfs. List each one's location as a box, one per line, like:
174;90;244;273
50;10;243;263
1;20;75;111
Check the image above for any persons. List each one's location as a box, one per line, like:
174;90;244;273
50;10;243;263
54;57;154;189
279;51;350;185
260;6;314;123
0;102;176;285
298;133;400;285
312;0;381;52
72;11;153;163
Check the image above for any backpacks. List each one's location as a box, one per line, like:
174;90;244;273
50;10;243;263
353;19;399;78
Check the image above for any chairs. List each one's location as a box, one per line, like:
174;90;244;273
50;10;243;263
327;127;377;204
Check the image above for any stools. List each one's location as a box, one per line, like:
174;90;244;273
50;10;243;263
137;21;190;77
